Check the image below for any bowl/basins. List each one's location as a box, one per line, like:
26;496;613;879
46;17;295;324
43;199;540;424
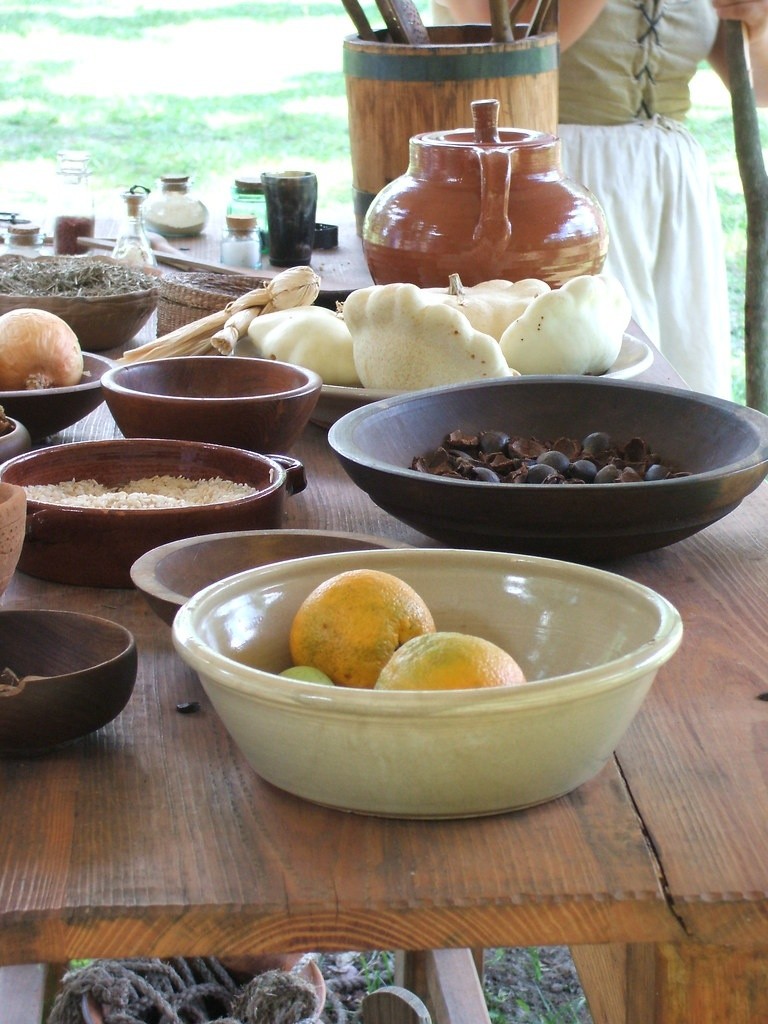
325;372;768;565
0;350;127;438
0;437;309;589
0;607;137;759
172;548;684;820
130;527;420;622
99;356;322;456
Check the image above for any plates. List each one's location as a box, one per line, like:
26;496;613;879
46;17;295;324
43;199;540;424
301;331;648;438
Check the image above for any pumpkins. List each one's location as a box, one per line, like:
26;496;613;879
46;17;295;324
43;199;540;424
0;307;84;392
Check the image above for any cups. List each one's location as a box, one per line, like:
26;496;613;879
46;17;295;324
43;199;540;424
260;169;318;267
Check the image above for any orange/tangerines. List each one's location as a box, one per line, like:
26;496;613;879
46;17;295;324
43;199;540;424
288;567;527;693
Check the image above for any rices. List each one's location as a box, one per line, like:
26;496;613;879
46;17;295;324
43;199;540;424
16;473;257;509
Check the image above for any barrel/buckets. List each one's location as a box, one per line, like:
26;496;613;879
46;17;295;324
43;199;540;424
343;22;559;247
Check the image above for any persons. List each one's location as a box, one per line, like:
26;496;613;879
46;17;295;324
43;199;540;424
439;0;768;399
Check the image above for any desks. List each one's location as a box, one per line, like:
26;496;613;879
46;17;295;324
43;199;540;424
0;321;768;1024
